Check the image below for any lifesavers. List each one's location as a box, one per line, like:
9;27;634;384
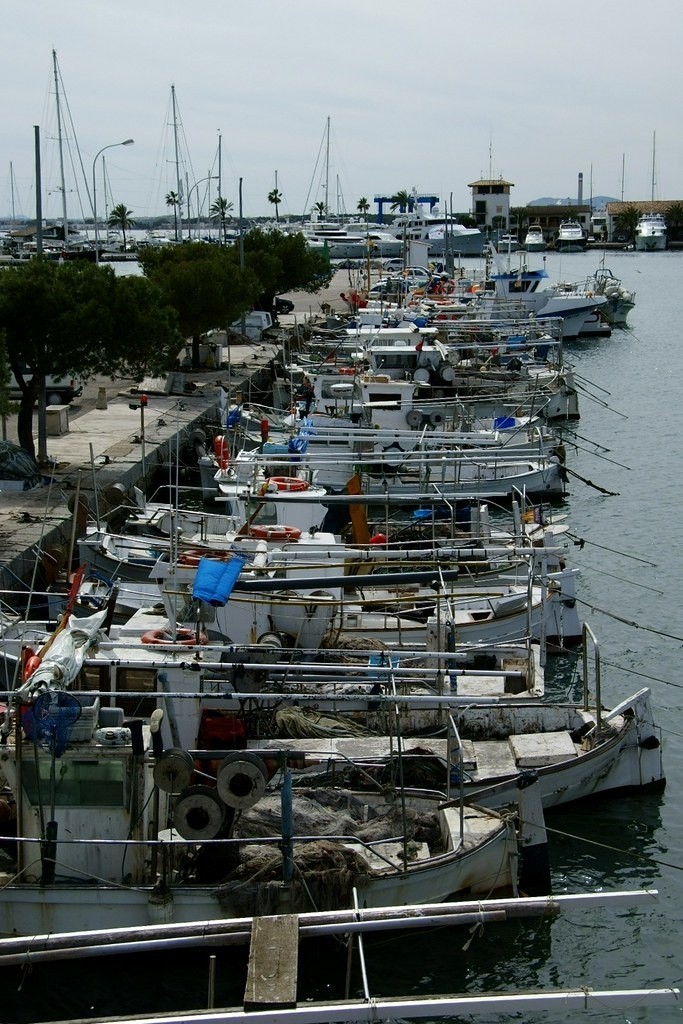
252;524;302;539
442;280;455;293
214;434;229;469
20;655;42;735
141;627;208;652
267;476;309;491
182;549;237;566
339;367;356;374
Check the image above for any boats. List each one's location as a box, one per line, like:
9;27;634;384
0;193;667;936
0;49;667;273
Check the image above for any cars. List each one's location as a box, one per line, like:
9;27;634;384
251;293;294;314
382;258;405;272
6;363;83;407
